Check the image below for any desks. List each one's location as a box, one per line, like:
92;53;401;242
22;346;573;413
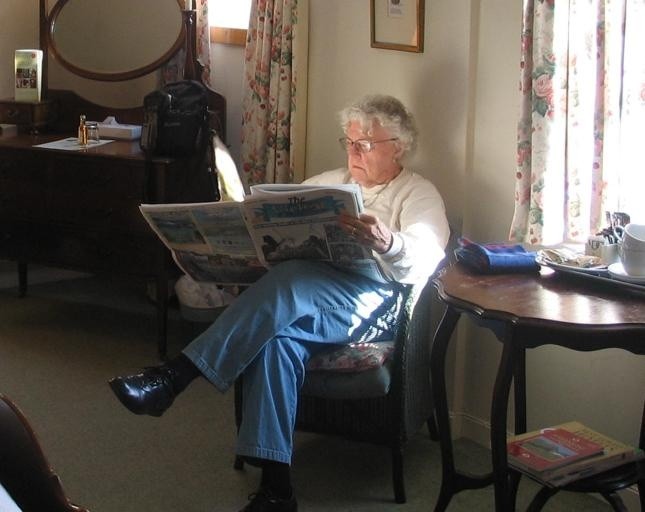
0;78;228;360
416;254;645;512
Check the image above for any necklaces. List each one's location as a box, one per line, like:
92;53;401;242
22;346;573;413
362;180;389;206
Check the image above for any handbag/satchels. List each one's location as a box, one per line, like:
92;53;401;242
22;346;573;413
139;79;226;151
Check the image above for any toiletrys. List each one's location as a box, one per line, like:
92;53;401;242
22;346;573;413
78;115;87;145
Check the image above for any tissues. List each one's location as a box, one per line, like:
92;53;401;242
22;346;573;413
84;116;143;141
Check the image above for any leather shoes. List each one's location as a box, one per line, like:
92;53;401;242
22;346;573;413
107;365;176;417
236;483;298;511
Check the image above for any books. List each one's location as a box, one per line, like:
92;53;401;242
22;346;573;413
507;421;645;489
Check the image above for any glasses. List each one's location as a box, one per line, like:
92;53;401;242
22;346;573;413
339;138;400;153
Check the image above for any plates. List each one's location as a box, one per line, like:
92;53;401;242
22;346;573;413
538;248;645;283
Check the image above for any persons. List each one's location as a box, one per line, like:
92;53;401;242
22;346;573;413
106;94;452;512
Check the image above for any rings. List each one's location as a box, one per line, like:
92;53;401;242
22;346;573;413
352;227;356;236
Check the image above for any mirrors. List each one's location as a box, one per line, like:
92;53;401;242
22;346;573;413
38;0;197;87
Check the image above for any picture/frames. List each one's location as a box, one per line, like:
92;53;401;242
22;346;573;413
367;0;426;54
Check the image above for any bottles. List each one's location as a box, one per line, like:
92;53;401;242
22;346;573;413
78;116;88;145
84;122;99;143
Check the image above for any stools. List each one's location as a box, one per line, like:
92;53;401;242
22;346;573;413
524;461;645;512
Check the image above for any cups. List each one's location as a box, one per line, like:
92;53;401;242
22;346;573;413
599;244;619;264
614;223;644;275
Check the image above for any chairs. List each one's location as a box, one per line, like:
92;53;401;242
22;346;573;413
1;395;89;511
221;222;462;504
